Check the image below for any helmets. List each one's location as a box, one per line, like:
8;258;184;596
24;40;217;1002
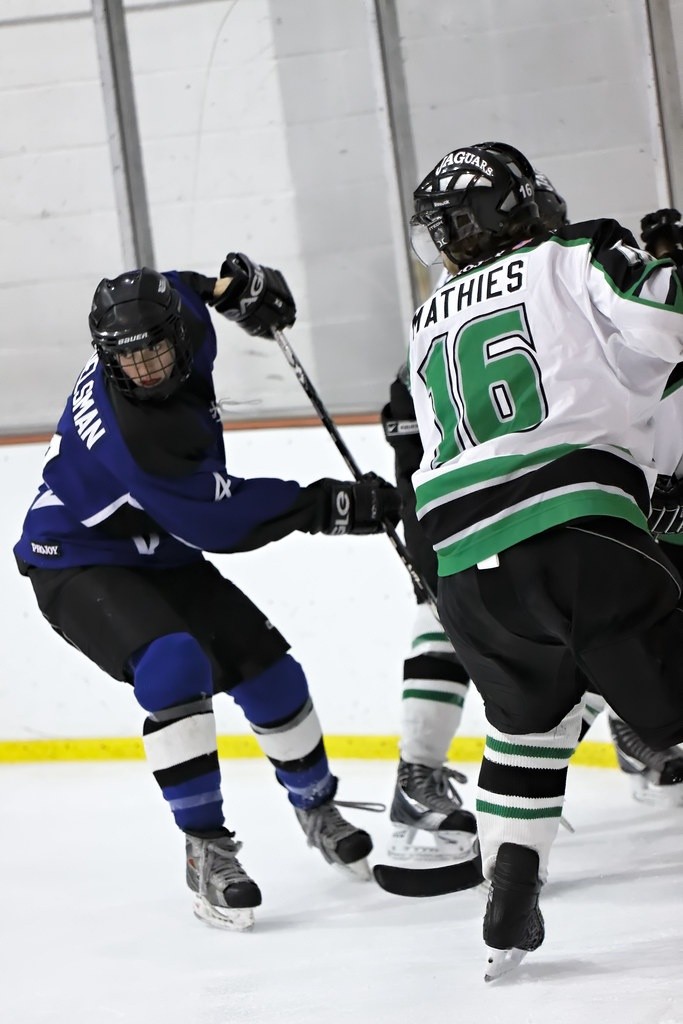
532;167;566;231
411;141;539;259
89;265;195;400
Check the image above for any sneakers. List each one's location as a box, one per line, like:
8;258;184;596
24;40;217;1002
607;716;683;806
184;831;263;931
386;759;478;862
293;797;387;883
482;842;547;984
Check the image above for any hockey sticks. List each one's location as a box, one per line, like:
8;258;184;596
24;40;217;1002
268;320;576;834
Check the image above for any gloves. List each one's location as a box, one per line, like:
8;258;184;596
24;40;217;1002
207;253;296;340
305;471;400;535
640;207;683;253
648;474;683;534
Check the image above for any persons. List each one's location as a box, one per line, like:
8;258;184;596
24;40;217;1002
13;255;402;934
406;142;683;982
380;168;683;862
